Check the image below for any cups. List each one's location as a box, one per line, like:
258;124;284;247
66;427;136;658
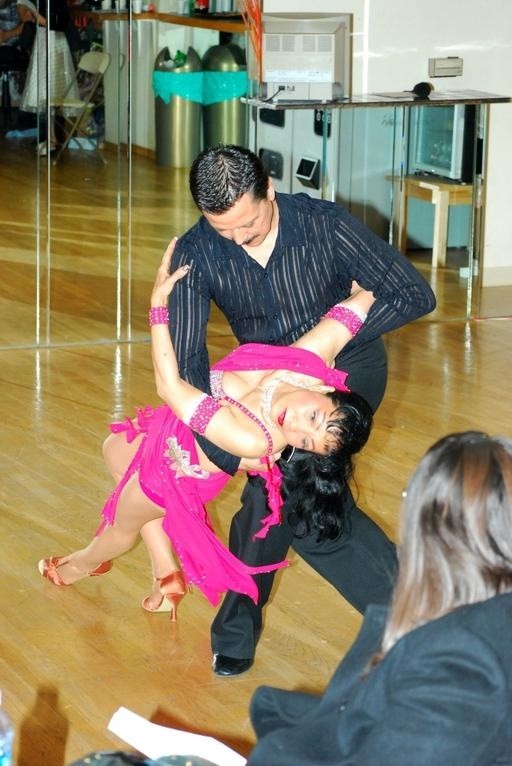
101;0;142;15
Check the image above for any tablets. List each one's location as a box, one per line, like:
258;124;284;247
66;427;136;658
294;157;318;180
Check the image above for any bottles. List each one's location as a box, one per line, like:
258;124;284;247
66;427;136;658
188;0;209;15
142;0;155;15
175;50;186;67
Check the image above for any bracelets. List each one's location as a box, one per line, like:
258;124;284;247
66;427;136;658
147;305;170;325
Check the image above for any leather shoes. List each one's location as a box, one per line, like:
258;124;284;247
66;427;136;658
210;655;254;678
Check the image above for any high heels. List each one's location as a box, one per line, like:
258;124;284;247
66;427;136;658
141;570;187;624
39;556;113;589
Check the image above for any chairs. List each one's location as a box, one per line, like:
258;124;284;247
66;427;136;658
41;51;112;165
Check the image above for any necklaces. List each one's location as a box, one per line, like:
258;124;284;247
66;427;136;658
262;375;315;434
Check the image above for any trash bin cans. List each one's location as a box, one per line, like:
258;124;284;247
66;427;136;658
154;43;246;167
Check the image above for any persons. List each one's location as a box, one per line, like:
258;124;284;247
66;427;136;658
20;0;80;157
37;234;377;621
0;0;27;71
243;428;512;766
163;145;438;677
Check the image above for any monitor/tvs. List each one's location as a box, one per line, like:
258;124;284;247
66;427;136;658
407;103;465;180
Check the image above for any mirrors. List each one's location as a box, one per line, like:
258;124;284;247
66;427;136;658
0;1;262;349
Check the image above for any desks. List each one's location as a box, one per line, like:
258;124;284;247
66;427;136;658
384;174;476;270
248;89;512;318
90;7;250;34
